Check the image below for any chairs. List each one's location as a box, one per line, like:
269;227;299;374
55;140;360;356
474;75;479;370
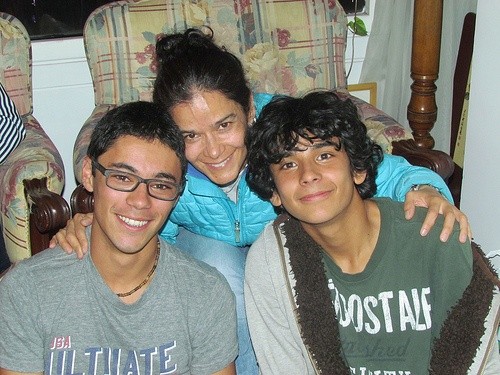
69;0;455;219
0;12;71;279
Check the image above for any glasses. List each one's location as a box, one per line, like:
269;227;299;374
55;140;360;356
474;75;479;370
91;159;185;201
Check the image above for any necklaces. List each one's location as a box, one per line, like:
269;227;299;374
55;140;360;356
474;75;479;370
116;238;160;297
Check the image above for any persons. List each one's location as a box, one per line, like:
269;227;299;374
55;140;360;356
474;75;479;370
50;24;471;375
244;86;500;375
0;102;239;375
